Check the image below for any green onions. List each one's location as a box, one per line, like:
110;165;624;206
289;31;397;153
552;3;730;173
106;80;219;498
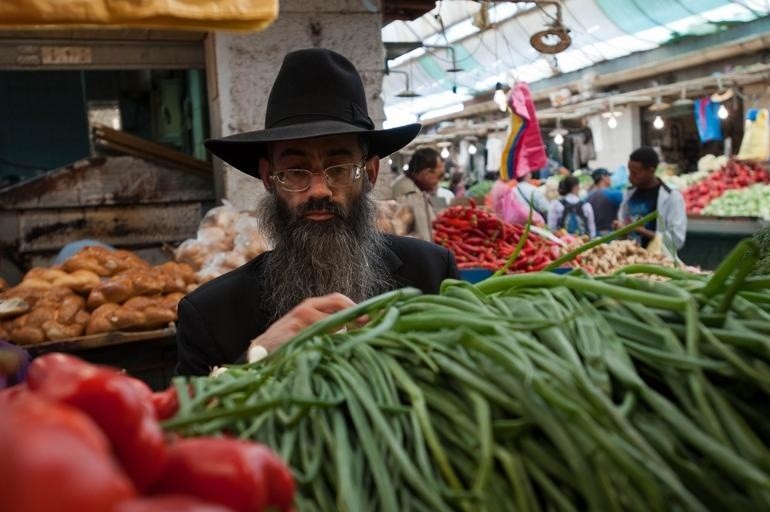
163;190;770;512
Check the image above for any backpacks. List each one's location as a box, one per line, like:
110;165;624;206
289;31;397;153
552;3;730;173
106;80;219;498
559;200;589;235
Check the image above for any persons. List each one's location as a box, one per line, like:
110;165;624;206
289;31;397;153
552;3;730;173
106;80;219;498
392;144;688;257
175;48;460;378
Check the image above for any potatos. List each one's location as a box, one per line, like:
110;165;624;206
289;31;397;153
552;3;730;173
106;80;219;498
0;245;216;344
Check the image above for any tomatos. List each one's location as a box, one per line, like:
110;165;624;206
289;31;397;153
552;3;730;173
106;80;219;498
1;352;297;511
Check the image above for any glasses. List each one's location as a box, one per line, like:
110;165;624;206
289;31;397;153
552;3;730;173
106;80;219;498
267;161;364;192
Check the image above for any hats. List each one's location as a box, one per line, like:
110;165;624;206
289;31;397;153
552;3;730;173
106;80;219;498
591;168;613;180
203;49;421;179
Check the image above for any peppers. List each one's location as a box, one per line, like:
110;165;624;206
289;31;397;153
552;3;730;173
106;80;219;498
431;197;594;276
